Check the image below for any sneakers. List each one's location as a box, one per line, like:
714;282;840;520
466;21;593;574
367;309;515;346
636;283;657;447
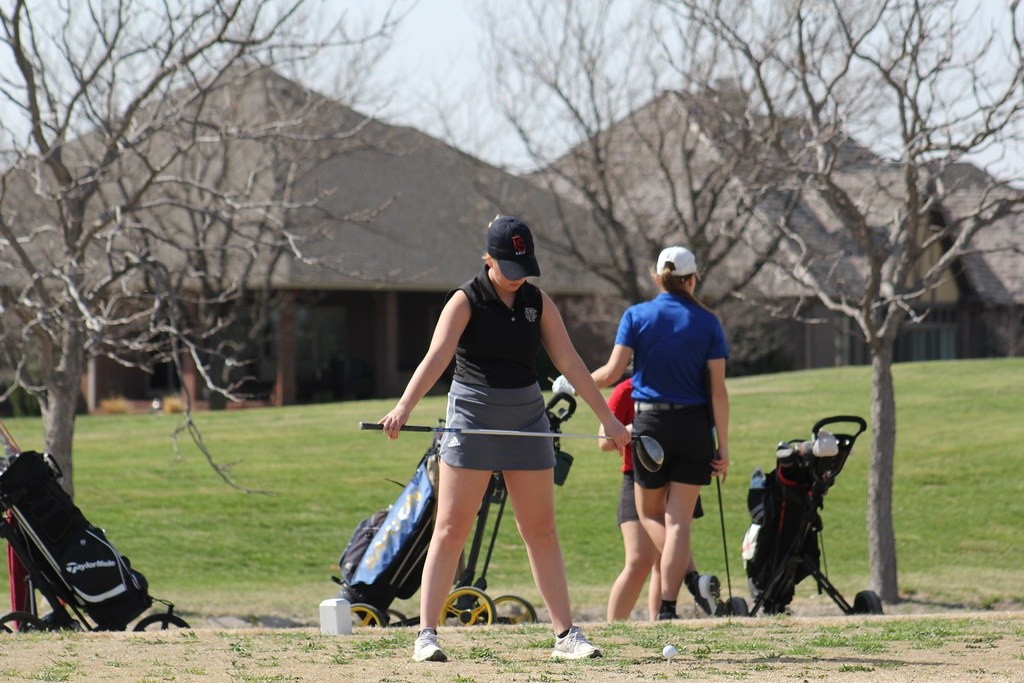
413;627;448;662
693;574;729;619
553;627;603;659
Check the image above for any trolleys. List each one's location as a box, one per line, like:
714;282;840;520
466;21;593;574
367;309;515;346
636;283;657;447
331;391;577;627
1;412;198;634
725;413;885;617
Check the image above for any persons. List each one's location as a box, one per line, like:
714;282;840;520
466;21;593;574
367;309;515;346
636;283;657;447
377;214;630;662
598;354;704;623
551;245;733;619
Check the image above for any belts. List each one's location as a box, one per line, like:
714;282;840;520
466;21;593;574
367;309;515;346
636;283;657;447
634;401;686;414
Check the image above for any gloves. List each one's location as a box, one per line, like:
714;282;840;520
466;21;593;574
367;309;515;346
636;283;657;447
551;373;576;396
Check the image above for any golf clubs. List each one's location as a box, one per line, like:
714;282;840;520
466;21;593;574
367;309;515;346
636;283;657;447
714;453;750;617
359;422;665;473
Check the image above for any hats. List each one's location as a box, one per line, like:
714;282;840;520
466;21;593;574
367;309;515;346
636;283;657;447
486;216;539;280
656;245;700;282
